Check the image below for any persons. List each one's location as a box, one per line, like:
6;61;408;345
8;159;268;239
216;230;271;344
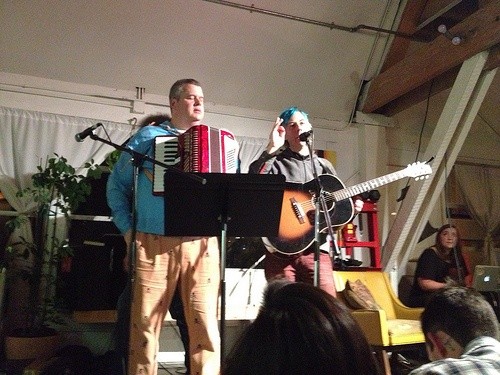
138;114;190;375
106;79;221;375
249;107;365;297
409;225;468;308
224;282;382;375
410;285;500;375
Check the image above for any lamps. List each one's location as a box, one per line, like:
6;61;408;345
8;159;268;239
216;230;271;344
437;24;461;46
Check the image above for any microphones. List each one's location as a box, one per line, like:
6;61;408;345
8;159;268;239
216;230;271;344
75;123;101;142
299;130;312;141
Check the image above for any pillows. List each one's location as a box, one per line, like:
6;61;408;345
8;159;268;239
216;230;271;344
344;279;384;311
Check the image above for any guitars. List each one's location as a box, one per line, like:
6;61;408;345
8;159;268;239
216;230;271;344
260;160;432;258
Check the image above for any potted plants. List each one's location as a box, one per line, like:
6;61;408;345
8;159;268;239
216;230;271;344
0;149;120;375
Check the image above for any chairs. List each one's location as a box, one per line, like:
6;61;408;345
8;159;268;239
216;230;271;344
331;270;427;375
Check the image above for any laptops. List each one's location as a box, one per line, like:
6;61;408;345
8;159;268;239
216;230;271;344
470;265;500;292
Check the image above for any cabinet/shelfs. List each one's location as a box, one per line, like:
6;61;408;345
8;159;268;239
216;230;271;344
330;200;383;270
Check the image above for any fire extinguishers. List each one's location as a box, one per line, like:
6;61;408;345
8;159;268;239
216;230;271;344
342;222;355;241
